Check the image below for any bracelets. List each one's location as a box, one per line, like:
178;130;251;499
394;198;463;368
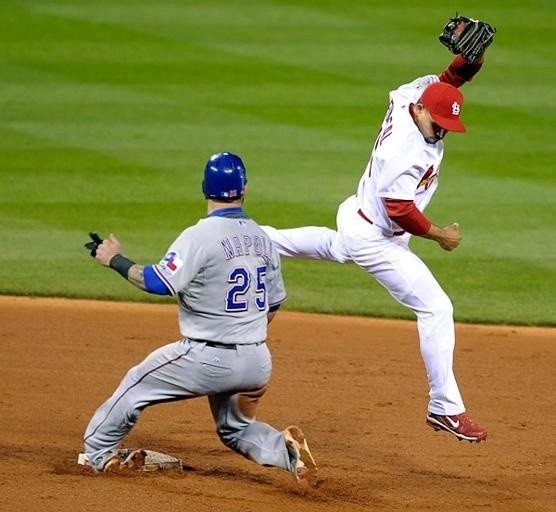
107;252;135;281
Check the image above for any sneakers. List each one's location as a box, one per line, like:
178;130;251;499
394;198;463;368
284;425;318;488
425;412;488;444
103;448;144;475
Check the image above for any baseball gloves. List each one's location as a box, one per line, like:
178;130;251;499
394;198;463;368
439;14;496;66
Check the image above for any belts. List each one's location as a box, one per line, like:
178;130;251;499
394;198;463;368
358;209;406;236
189;339;234;350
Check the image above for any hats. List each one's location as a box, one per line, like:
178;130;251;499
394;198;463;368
420;84;466;133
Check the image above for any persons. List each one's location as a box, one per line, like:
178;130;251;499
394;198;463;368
253;9;500;446
76;149;320;494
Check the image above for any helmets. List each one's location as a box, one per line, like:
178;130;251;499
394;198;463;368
203;151;247;200
